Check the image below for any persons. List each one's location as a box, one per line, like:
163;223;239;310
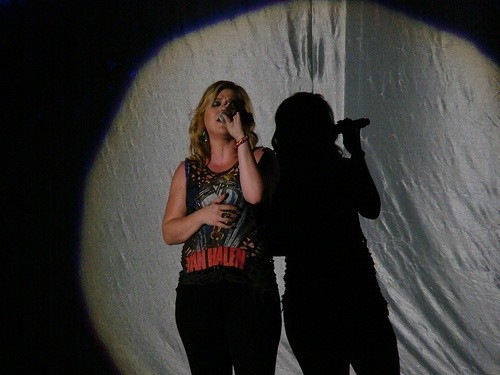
162;81;282;375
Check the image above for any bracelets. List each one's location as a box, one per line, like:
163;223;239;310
234;136;250;148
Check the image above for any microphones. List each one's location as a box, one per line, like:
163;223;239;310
218;96;245;122
